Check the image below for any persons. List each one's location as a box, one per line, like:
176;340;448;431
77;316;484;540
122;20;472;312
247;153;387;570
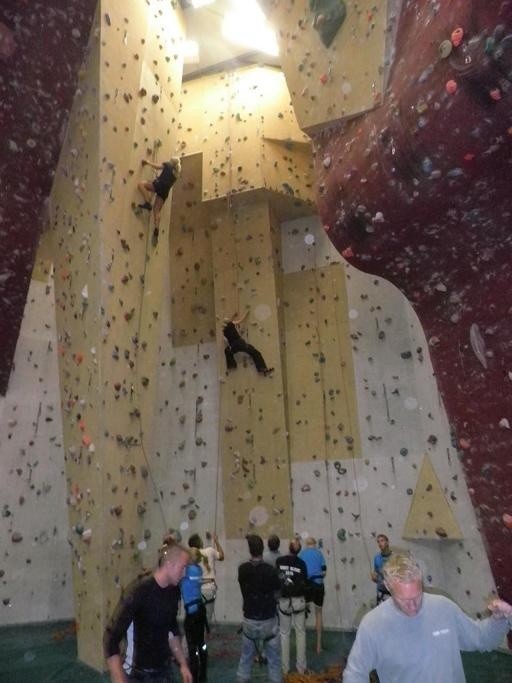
188;532;225;640
118;618;136;675
371;534;397;604
274;539;309;674
157;526;189;656
222;310;274;374
263;534;281;566
297;537;326;652
137;156;180;235
237;534;284;682
102;542;196;682
341;548;512;681
180;547;210;683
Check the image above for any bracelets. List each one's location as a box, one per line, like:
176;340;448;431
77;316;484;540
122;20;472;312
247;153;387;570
171;646;178;653
